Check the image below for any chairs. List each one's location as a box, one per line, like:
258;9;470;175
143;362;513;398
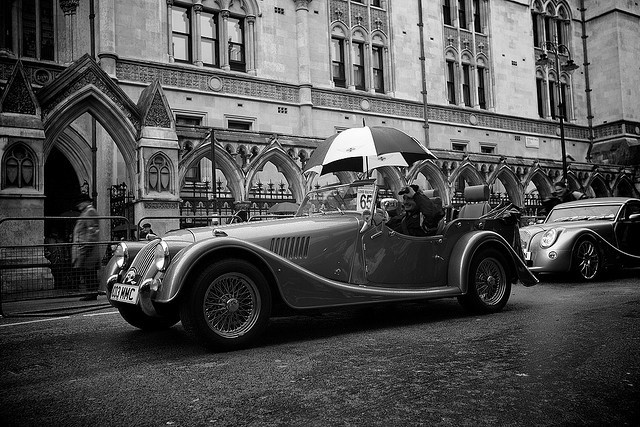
457;184;491;219
424;189;442;206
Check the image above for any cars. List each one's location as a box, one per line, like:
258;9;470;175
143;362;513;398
521;195;640;284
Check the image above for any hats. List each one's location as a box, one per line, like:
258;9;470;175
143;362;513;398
73;194;92;206
142;224;151;227
398;185;423;196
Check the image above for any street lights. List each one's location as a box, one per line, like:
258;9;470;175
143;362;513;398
536;34;579;178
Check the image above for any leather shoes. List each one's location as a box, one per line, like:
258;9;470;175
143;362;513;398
79;294;96;301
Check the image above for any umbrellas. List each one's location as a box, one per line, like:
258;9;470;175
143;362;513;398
301;118;438;179
110;222;143;231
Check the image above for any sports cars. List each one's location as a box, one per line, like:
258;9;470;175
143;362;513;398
108;177;538;354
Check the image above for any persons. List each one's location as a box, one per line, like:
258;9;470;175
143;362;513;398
70;193;100;301
105;234;119;260
384;185;445;237
139;224;156;239
541;181;577;215
